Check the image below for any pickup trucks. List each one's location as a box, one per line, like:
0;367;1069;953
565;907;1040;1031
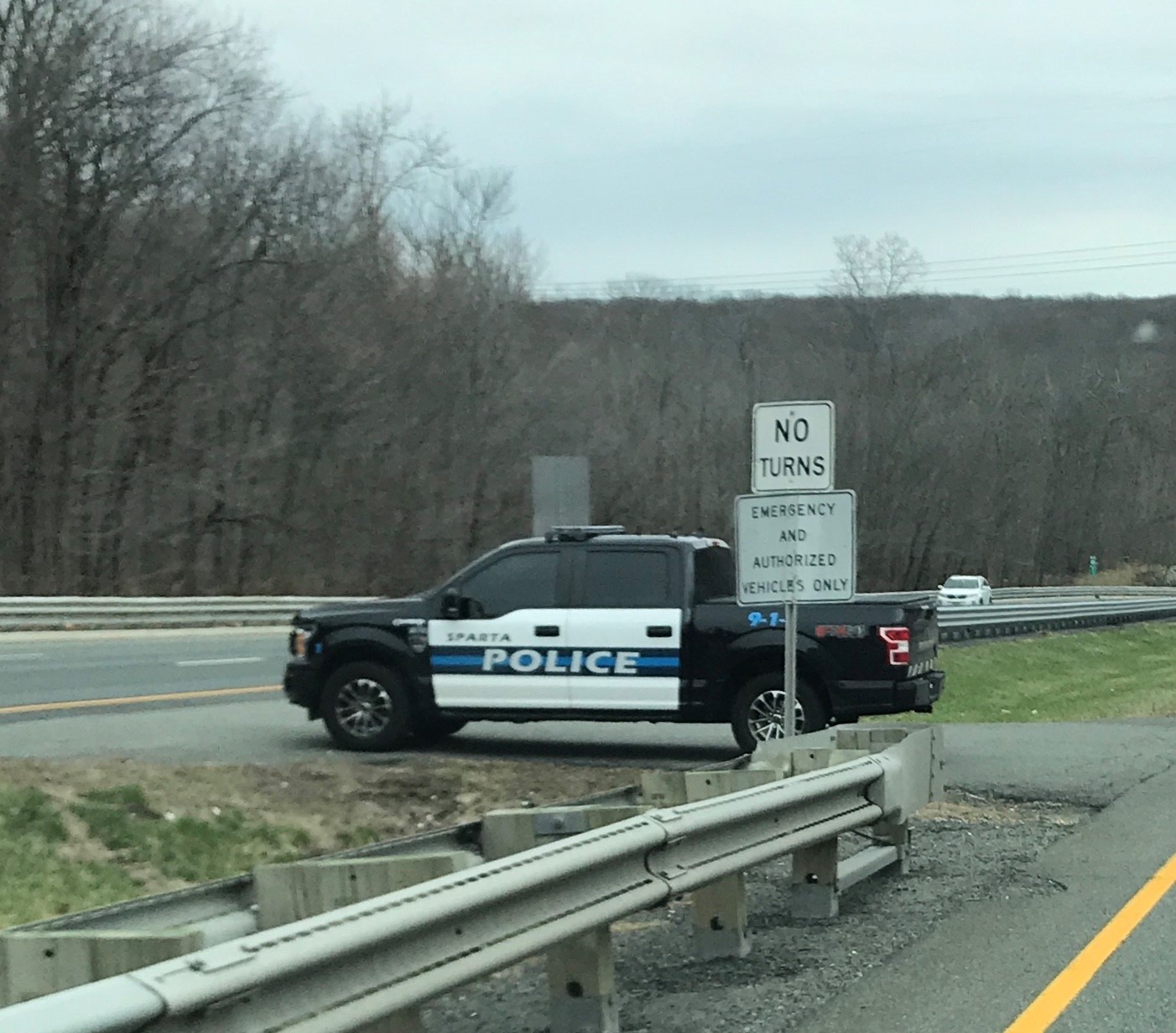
283;524;947;757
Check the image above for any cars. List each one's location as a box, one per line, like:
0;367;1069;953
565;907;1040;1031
936;575;992;606
